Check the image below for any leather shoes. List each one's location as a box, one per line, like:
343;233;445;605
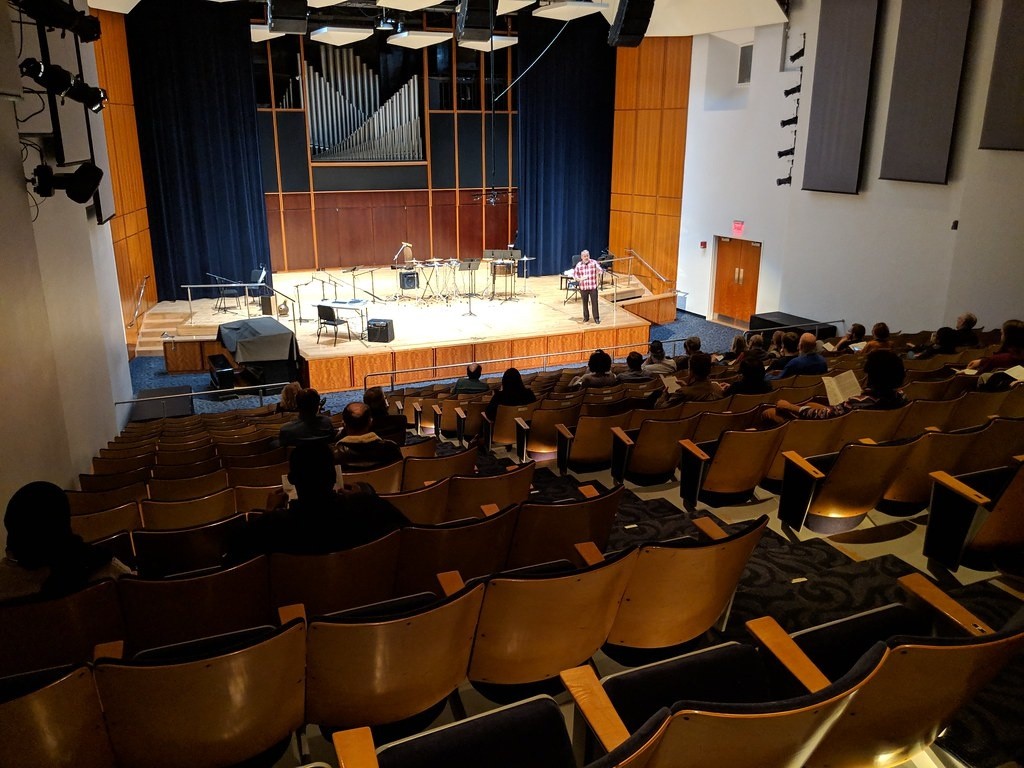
584;318;589;322
595;319;599;324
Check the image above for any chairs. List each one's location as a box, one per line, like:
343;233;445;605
0;326;1024;768
560;255;582;302
244;270;268;306
215;278;241;313
317;305;351;347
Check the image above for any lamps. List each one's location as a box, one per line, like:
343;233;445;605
474;0;516;205
377;7;394;31
8;0;102;44
19;57;108;114
776;48;805;186
33;161;103;204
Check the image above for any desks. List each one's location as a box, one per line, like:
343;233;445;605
311;302;368;340
129;385;193;422
747;312;837;345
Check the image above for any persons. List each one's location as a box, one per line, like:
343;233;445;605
916;327;957;360
247;441;410;554
279;388;337;446
814;324;865;356
861;322;895;352
275;382;326;412
363;386;407;436
574;250;603;324
455;363;490;394
906;312;978;352
479;368;536;452
0;481;130;599
766;333;828;380
760;349;910;429
568;330;783;409
968;320;1024;368
335;402;402;462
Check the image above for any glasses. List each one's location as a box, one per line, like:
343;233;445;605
748;340;754;343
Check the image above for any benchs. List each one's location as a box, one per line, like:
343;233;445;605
207;353;239;401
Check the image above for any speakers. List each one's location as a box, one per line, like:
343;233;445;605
455;0;499;42
260;296;277;315
368;319;394;343
267;0;308;34
400;272;419;289
607;0;655;48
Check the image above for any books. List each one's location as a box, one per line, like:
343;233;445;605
823;342;834;351
849;342;867;352
999;365;1024;387
950;367;978;374
821;370;862;406
